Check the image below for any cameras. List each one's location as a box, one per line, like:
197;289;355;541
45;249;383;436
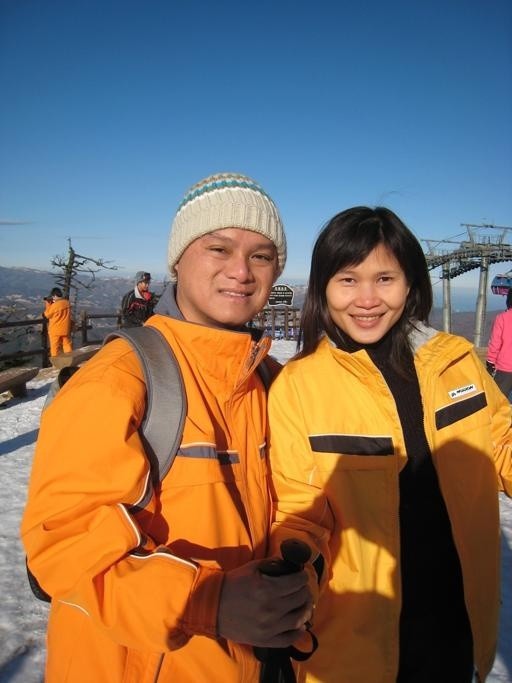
44;296;53;304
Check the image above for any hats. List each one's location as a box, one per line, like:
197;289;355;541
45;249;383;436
135;270;151;285
168;172;288;284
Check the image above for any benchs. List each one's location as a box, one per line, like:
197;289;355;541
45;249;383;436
49;343;100;370
0;365;41;399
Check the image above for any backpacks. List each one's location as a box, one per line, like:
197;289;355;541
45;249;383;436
25;325;274;606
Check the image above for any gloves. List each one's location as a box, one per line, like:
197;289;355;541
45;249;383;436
487;366;496;378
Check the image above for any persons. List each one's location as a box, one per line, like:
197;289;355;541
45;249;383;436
43;287;72;366
486;286;512;407
263;206;512;683
121;269;157;329
18;174;311;683
